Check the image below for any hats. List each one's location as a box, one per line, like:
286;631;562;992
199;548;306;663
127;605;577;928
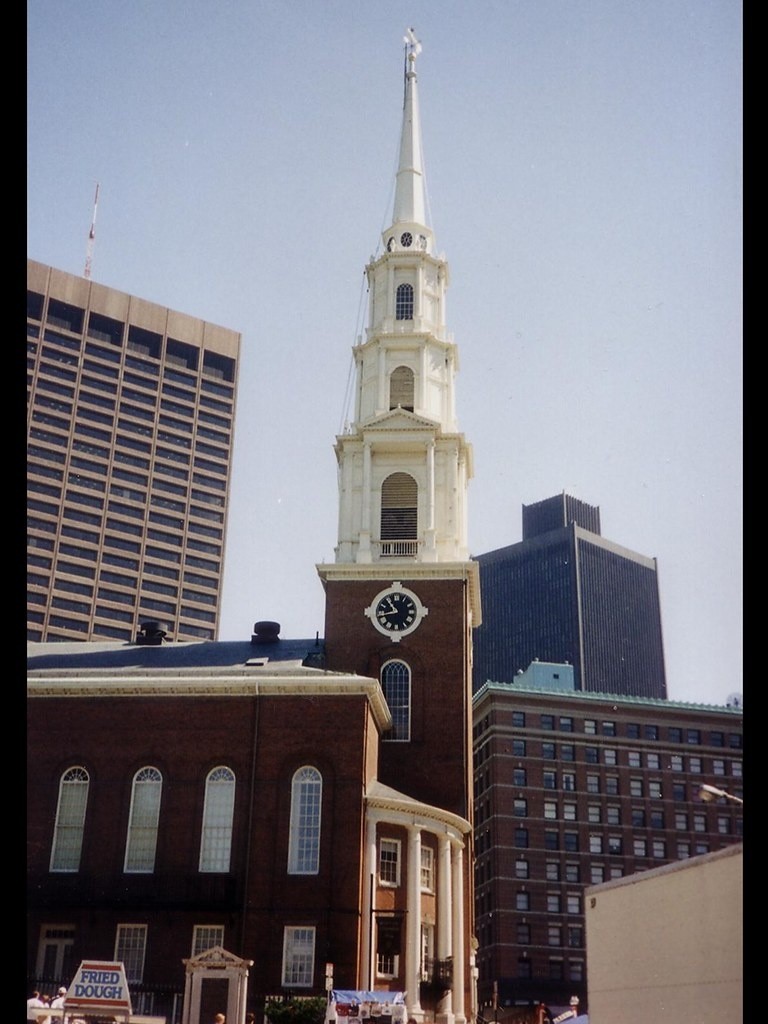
59;987;67;994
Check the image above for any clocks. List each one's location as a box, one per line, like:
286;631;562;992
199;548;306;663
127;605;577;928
363;582;428;642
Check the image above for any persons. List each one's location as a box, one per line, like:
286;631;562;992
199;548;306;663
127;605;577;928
214;1013;226;1024
27;986;67;1024
